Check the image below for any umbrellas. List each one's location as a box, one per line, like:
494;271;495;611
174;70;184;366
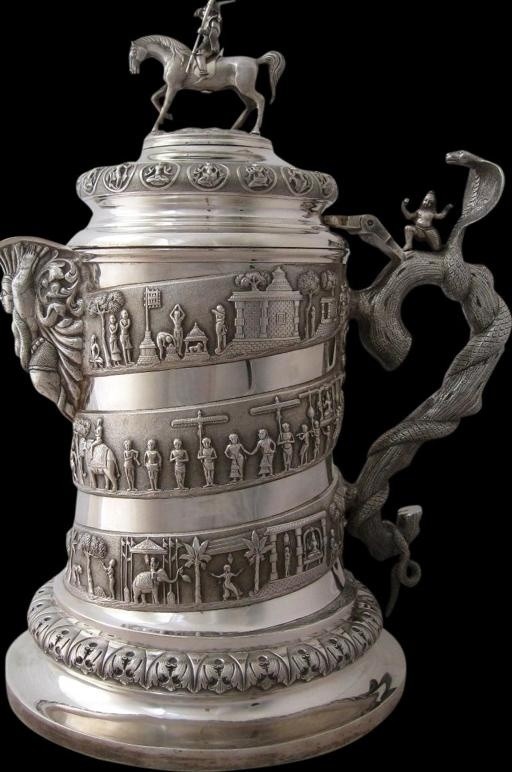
129;538;169;559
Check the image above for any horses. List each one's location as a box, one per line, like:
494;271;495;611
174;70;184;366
129;33;286;135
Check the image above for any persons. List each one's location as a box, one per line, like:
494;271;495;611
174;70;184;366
398;188;453;254
194;5;223;78
89;303;228;369
86;404;324;492
149;554;159;579
209;564;245;601
306;534;321;558
284;546;292;577
99;556;117;600
